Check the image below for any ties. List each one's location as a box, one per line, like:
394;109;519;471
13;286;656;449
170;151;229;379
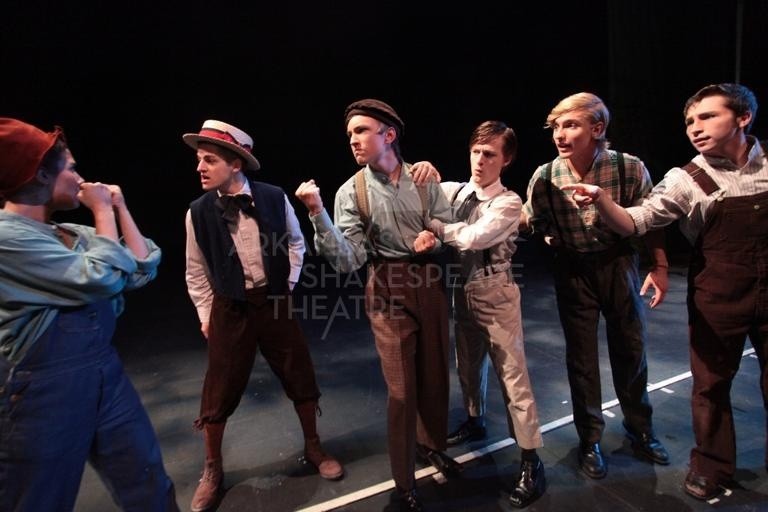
456;191;481;223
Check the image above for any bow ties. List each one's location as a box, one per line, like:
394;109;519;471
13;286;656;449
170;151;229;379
213;194;259;225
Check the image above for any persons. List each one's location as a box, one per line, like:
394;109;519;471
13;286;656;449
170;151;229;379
179;110;350;510
408;117;552;507
292;98;469;510
1;113;180;512
562;82;768;500
513;91;672;478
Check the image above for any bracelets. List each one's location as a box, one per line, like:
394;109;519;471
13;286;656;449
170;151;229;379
653;263;669;271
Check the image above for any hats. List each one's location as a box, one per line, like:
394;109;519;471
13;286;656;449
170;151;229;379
0;117;63;197
183;119;260;171
344;98;404;140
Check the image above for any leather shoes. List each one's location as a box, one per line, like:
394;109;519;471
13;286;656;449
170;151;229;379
305;435;343;479
390;419;545;512
579;439;606;479
190;455;224;512
623;418;671;465
684;470;721;500
